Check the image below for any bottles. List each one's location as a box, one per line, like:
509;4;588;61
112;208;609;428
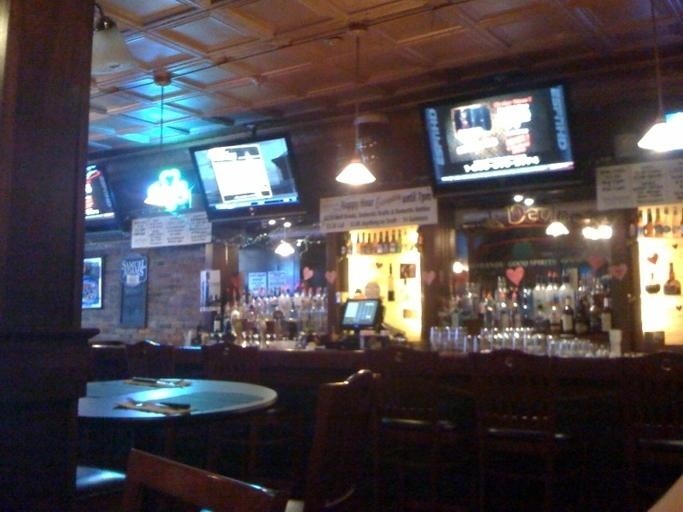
387;264;394;301
213;284;327;347
445;273;612;339
356;229;403;255
639;206;682;296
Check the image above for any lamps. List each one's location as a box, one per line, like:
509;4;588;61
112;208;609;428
334;21;377;187
90;2;141;77
142;70;192;211
632;1;682;156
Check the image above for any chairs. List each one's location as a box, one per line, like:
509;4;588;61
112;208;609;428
67;323;682;511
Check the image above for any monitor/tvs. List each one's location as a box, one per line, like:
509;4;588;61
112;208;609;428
189;132;311;222
85;164;122;233
420;80;579;193
340;299;381;327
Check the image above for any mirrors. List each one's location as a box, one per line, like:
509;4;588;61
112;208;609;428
237;233;325;300
452;214;617;314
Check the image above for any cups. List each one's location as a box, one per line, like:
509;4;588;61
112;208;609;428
430;321;621;360
